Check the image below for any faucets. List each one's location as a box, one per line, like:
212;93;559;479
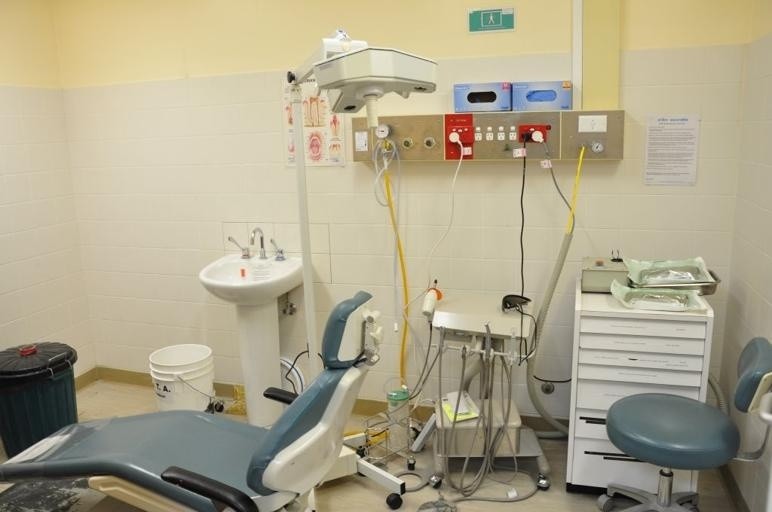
250;228;266;259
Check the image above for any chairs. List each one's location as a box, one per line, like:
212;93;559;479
597;334;772;512
0;290;382;512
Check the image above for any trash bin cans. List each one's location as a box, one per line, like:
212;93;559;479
0;342;77;460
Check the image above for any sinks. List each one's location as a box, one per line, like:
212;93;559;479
199;253;301;303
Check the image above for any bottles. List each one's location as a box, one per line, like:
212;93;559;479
388;392;410;449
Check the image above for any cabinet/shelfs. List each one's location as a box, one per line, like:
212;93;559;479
565;276;715;496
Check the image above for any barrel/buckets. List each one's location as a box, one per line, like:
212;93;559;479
149;343;217;414
